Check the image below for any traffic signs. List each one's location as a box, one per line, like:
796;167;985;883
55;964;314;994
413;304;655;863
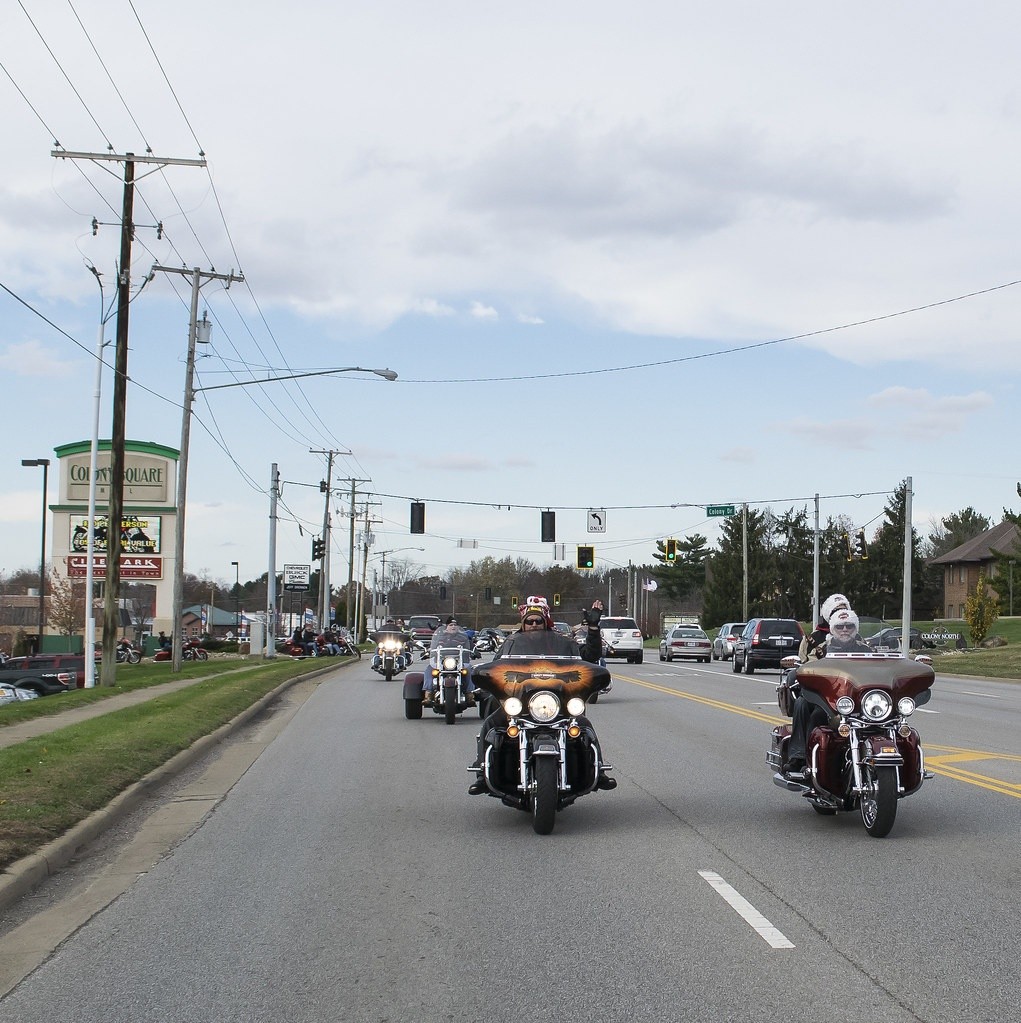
284;563;311;592
587;510;606;533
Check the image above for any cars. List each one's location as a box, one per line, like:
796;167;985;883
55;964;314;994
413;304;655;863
274;637;290;652
216;636;250;645
659;628;713;663
551;622;571;636
863;626;937;651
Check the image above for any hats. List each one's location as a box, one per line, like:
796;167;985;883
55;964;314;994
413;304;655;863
827;608;859;643
446;615;457;625
386;617;393;622
519;596;555;631
822;593;852;623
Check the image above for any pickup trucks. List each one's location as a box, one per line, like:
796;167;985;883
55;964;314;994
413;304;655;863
0;657;78;697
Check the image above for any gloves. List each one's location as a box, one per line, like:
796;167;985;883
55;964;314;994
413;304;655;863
420;652;429;661
470;651;482;661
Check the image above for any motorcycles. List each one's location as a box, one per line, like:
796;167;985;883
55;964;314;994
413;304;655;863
153;635;208;663
765;617;935;838
284;632;330;659
470;632;614;836
368;627;416;681
402;624;486;726
72;638;142;664
562;632;620;694
315;627;362;659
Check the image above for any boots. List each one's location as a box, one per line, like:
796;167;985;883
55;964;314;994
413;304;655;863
422;690;433;706
464;692;477;708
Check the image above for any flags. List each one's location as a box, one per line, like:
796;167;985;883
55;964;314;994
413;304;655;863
306;608;313;624
643;580;657;592
331;607;336;620
238;610;247;633
201;606;206;626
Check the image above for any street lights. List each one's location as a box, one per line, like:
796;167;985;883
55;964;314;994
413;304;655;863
171;366;400;664
380;547;426;606
231;561;239;637
21;457;51;653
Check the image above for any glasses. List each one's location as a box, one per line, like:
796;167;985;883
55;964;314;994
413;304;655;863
836;624;855;630
830;608;839;617
524;618;544;625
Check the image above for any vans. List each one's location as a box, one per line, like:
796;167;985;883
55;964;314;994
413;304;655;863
2;656;100;689
669;623;702;630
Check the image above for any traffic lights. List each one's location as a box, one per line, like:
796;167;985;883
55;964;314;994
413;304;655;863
577;546;594;568
312;539;319;561
619;592;625;605
666;539;677;561
840;536;851;561
554;594;560;606
855;532;867;557
382;593;388;606
512;597;518;609
318;538;326;560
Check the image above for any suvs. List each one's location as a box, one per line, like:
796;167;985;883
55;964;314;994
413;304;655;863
731;617;806;675
712;622;747;661
404;616;442;646
598;616;644;663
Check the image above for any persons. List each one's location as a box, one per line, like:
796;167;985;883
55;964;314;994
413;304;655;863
468;595;617;794
421;616;475;703
293;624;319;656
386;618;405;632
781;593;879;771
158;631;172;651
322;624;345;656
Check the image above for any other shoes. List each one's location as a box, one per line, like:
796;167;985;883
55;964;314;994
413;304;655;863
371;664;378;669
399;666;408;670
787;757;808;773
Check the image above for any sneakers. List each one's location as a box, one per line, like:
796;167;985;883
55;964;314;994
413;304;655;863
468;775;489;794
597;771;618;791
